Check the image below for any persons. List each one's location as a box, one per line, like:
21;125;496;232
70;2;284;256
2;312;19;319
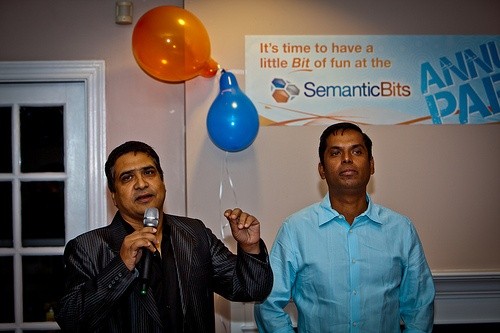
51;141;273;333
254;122;436;333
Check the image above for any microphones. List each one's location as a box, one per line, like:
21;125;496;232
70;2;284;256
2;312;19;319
140;207;160;295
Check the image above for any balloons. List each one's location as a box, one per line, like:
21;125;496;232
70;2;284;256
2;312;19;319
132;6;218;82
207;70;259;152
